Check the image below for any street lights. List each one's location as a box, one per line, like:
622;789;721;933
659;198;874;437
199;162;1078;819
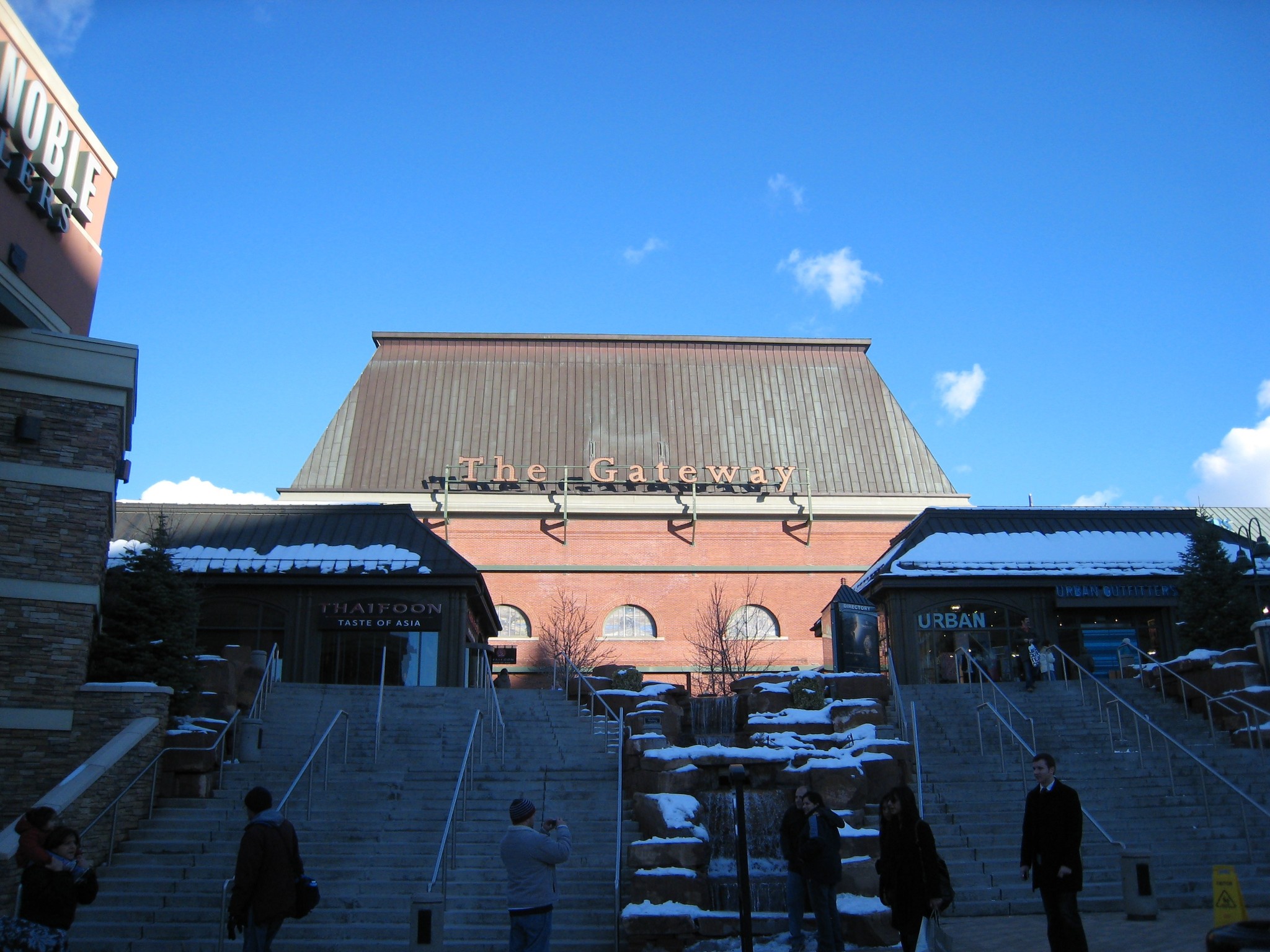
1234;516;1270;621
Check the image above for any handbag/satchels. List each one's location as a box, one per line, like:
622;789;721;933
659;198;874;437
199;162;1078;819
290;876;320;919
0;916;68;952
915;909;953;952
1028;641;1041;668
922;858;952;918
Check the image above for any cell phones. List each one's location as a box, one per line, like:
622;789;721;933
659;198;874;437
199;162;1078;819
550;820;557;826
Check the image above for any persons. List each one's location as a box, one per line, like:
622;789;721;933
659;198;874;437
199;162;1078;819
1006;615;1055;690
229;786;304;952
15;806;99;952
1020;754;1088;952
780;784;844;952
875;786;936;952
493;669;511;689
501;799;571;952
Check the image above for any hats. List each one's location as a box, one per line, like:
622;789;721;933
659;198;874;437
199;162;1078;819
245;787;273;812
511;798;536;824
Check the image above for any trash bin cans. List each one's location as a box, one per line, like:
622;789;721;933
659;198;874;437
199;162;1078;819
409;894;444;949
1119;848;1160;922
1120;655;1133;666
239;718;264;762
251;650;268;668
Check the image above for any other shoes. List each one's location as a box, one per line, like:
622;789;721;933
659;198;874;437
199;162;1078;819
789;943;805;952
1027;686;1033;692
816;944;833;952
1033;682;1036;688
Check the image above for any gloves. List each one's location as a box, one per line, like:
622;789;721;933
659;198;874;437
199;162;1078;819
227;916;243;940
